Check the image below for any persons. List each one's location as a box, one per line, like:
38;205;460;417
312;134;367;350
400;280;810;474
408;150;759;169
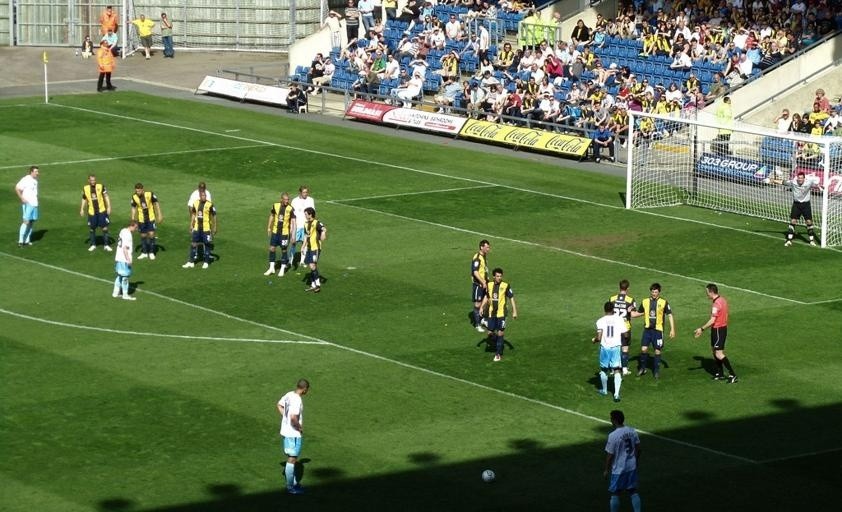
603;411;643;512
277;379;311;495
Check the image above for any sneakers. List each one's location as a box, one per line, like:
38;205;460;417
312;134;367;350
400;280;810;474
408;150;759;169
589;365;738;404
286;487;306;496
476;325;485;333
182;262;195;268
137;252;148;259
492;353;502;363
17;243;33;249
263;268;275;276
784;241;792;248
87;244;96;252
809;241;817;247
149;252;155;261
103;244;113;252
111;293;137;301
277;269;285;277
201;262;209;269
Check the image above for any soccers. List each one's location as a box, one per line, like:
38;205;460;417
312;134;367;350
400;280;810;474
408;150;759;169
482;470;496;482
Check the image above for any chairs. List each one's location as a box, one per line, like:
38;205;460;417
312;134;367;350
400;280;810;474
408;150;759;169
293;4;527;103
577;3;840;95
445;61;704;143
